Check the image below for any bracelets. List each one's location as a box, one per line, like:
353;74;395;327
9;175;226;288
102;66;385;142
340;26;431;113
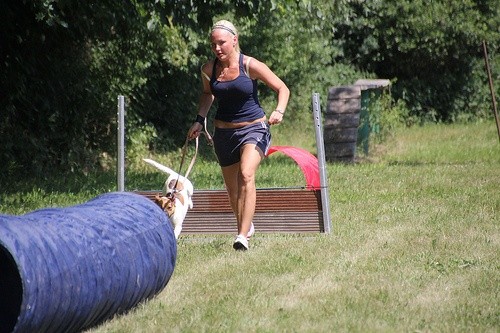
275;109;284;116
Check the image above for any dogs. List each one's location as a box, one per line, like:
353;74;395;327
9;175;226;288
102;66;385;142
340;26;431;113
142;159;193;239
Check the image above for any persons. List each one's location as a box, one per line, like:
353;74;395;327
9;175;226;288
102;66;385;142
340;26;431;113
187;19;290;251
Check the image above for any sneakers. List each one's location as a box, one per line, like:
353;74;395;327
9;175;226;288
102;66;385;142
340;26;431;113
233;235;248;250
246;222;254;240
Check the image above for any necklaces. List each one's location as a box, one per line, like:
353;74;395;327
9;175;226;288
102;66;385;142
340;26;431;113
218;62;232;77
